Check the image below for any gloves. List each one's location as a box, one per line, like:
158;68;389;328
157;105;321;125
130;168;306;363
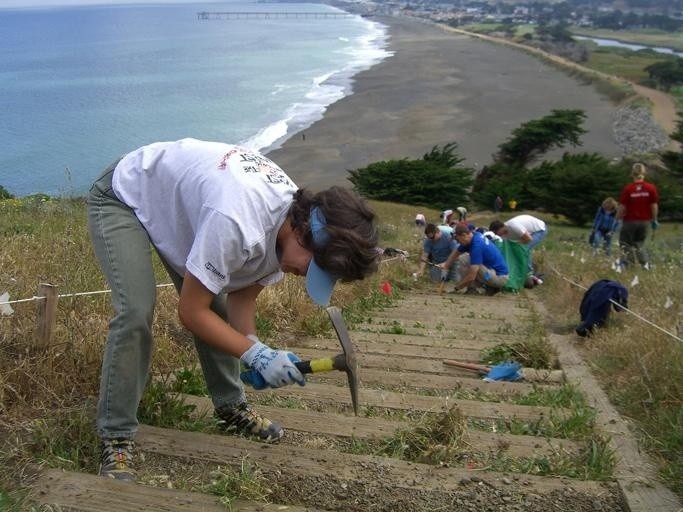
611;221;620;232
240;334;305;390
651;220;659;230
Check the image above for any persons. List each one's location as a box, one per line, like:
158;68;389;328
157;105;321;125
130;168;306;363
494;196;503;213
422;213;548;294
588;197;621;258
439;209;454;224
454;206;467;222
84;137;382;486
508;197;518;213
415;213;426;226
616;162;659;271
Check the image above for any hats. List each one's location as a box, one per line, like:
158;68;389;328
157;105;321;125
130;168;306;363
304;205;345;307
630;163;645;177
468;224;475;229
451;222;470;234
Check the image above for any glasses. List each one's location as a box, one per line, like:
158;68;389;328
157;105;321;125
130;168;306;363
430;233;435;239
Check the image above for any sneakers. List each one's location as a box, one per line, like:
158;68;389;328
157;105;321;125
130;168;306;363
102;438;134;481
464;288;479;294
485;284;499;296
214;404;284;443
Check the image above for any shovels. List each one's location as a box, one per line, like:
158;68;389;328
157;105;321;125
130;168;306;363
443;359;522;380
